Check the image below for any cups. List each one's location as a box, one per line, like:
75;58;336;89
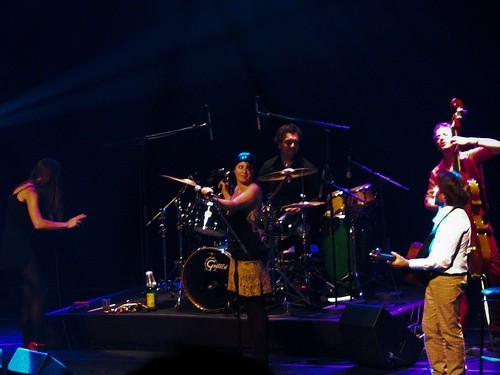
101;299;111;313
145;271;157;287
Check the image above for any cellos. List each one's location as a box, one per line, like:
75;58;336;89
449;97;493;341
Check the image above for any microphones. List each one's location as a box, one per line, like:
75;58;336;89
208;111;214;138
344;152;353;180
256;101;263;127
317;169;326;198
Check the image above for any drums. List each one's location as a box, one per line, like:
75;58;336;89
192;200;228;237
325;183;376;219
180;240;243;313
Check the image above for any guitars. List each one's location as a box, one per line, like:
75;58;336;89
368;240;429;289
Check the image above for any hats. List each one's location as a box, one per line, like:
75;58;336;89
233;152;258;167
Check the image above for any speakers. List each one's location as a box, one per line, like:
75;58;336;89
339;303;425;369
6;346;76;375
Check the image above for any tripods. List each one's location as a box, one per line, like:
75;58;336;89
140;174;343;308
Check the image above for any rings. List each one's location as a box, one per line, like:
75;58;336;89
474;137;479;147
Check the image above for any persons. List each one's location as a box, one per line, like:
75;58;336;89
0;155;88;352
260;120;321;299
389;170;473;375
422;122;500;342
196;148;273;364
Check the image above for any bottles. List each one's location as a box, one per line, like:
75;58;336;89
146;287;158;310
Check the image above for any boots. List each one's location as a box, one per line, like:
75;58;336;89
240;303;270;364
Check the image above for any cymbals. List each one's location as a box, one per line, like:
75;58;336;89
282;201;325;209
159;172;198;188
257;166;320;182
